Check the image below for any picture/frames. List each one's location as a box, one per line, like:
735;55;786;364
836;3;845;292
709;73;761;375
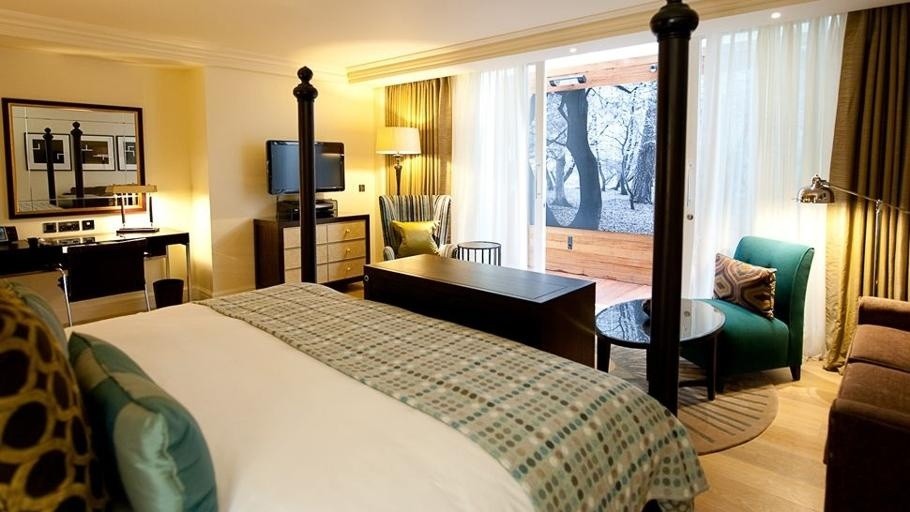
24;132;72;171
80;134;116;171
116;135;137;171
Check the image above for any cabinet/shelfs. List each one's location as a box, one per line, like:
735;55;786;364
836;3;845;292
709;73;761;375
253;214;370;289
362;254;597;369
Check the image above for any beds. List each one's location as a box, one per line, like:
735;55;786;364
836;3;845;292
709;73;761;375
61;282;710;511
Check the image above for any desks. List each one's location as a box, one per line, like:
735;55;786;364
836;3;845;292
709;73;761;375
0;229;191;302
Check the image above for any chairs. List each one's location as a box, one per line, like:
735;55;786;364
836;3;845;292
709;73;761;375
680;236;815;394
378;194;458;261
54;238;151;327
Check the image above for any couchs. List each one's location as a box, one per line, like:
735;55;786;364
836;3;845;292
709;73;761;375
822;295;910;512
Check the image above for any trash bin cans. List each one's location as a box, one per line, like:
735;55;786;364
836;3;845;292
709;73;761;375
153;279;184;309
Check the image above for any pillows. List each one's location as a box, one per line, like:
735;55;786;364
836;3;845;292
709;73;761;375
713;253;780;320
67;330;218;512
392;220;439;258
0;300;109;512
5;279;68;355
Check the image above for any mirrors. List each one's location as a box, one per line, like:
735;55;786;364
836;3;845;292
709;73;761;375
2;98;147;220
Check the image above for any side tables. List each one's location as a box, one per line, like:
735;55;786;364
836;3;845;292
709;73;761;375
458;241;501;266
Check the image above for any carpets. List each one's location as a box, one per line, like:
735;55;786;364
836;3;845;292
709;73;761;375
594;340;778;454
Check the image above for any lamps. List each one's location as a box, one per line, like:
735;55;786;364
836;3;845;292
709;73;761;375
797;175;910;296
376;127;422;195
105;184;160;233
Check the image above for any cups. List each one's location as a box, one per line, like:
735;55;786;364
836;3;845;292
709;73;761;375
28;237;40;248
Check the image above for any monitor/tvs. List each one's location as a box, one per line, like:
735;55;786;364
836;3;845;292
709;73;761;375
267;140;345;194
